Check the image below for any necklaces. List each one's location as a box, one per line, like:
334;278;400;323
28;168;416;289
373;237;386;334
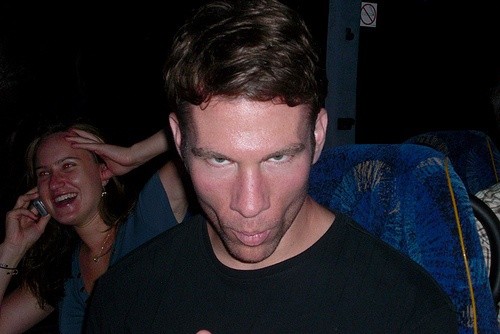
82;217;120;261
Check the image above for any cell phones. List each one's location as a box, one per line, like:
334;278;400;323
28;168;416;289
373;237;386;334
33;198;48;217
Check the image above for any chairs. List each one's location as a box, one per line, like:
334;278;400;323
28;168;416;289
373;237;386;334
405;129;500;195
311;145;499;333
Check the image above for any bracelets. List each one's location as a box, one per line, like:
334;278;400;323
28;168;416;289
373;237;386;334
0;263;17;272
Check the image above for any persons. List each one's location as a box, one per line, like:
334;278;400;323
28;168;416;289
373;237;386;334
0;120;196;334
83;0;471;334
